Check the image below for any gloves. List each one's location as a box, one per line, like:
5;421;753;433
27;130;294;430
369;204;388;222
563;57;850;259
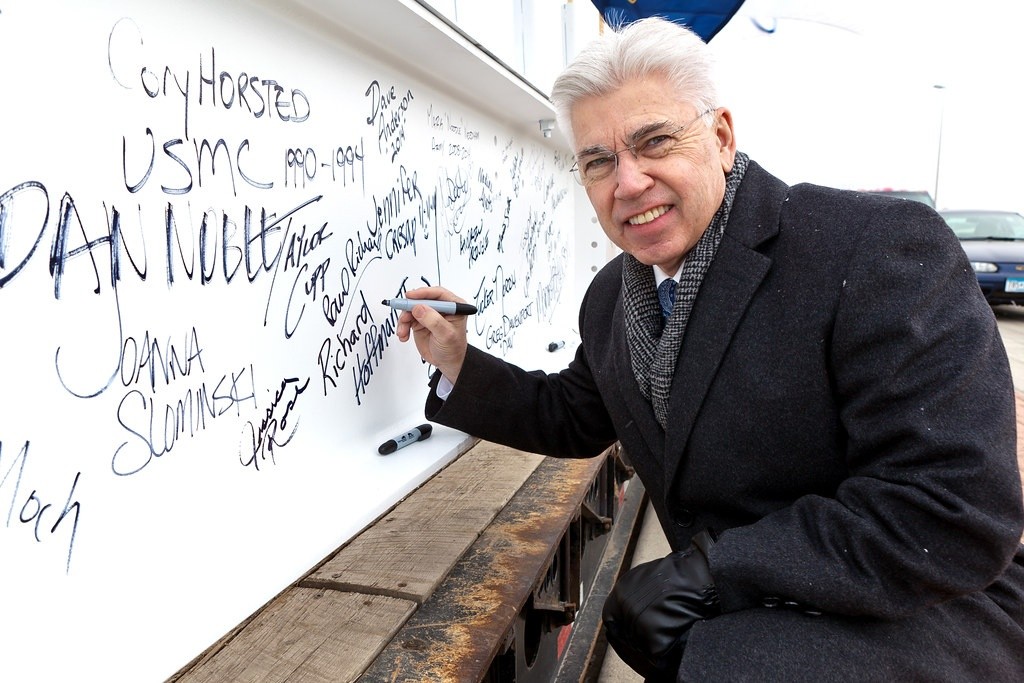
602;531;723;681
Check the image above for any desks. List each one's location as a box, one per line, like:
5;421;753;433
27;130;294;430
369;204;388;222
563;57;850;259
168;442;651;683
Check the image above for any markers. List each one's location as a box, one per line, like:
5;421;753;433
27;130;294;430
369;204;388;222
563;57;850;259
378;424;432;455
381;298;478;316
549;341;565;352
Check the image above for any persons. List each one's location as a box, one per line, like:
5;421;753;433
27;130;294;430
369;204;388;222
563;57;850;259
395;16;1024;683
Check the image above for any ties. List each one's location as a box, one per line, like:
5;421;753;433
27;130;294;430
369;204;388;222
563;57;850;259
658;279;678;320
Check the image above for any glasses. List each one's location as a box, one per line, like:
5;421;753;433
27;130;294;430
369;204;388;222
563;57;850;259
569;109;715;186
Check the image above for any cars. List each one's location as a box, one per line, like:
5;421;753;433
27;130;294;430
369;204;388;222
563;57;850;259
934;208;1024;310
863;188;935;210
